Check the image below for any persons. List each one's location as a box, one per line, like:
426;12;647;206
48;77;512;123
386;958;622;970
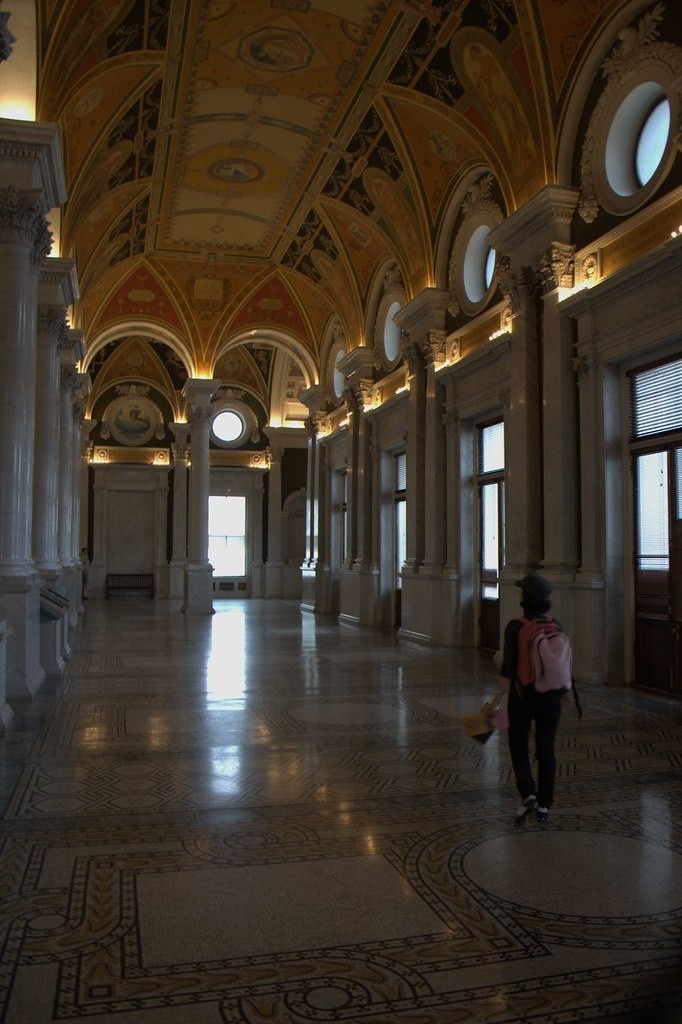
481;574;564;825
79;548;90;599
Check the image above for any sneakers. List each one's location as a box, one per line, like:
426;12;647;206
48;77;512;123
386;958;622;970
538;807;549;822
512;794;537;828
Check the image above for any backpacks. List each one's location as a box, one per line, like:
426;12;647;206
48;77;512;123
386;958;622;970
514;614;572;700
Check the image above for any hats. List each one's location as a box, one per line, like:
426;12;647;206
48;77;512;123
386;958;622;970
516;574;553;594
